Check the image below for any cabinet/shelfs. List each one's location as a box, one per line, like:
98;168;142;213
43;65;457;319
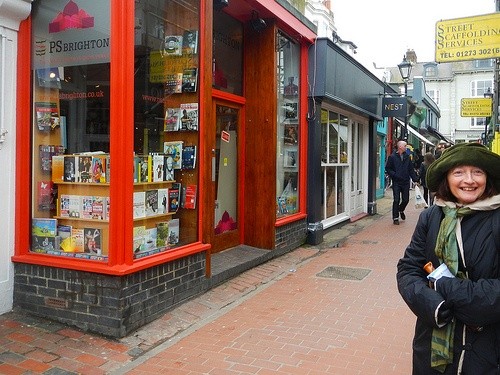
52;151;177;256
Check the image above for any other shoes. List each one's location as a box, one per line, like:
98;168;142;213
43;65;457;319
399;210;406;220
393;219;399;225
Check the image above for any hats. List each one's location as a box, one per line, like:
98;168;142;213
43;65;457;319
426;142;500;196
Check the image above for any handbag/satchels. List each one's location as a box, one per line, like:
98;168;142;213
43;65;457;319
414;184;428;209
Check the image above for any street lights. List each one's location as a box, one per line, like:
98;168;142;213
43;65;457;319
483;87;493;145
397;54;413;147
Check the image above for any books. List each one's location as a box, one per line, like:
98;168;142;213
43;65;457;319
163;30;198;56
34;101;61;131
31;141;197;263
164;68;198;94
163;103;197;133
282;75;298;197
37;68;62;89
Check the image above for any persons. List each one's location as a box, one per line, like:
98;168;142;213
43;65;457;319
396;142;500;375
412;142;451;209
166;37;179;53
289;127;297;143
185;32;195;48
291;152;295;166
284;77;298;95
162;195;166;213
79;157;89;172
166;156;175;181
385;140;419;225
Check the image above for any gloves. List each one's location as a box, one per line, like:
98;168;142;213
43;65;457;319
437;302;454;322
